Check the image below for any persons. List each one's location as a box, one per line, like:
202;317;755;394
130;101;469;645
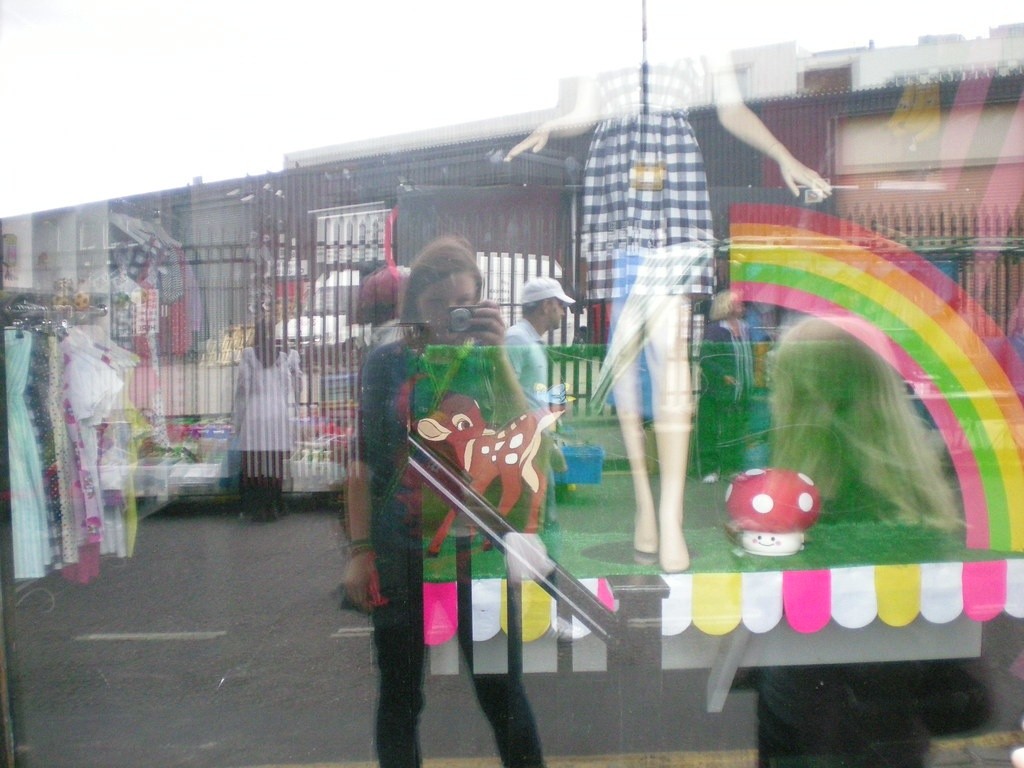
229;323;303;524
340;236;546;767
724;322;993;767
699;290;770;484
501;276;577;643
501;32;833;572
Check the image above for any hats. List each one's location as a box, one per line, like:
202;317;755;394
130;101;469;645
520;276;577;306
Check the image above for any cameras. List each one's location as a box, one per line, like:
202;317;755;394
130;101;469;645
447;305;488;332
